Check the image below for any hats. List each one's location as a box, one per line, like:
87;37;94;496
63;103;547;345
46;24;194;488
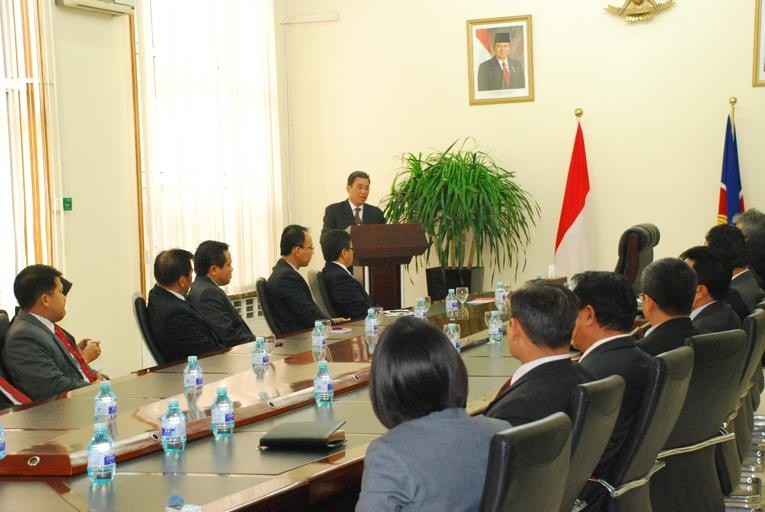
494;34;510;43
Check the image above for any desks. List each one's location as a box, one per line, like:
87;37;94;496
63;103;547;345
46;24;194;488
0;291;646;511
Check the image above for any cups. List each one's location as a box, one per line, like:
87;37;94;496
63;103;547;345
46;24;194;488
443;324;460;341
483;309;503;328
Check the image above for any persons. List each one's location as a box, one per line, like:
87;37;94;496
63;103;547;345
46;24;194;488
680;245;743;334
1;264;112;402
705;224;765;313
319;229;380;322
264;225;351;335
486;284;594;431
734;207;765;277
146;249;227;363
319;170;386;275
188;240;257;348
478;33;525;91
568;270;655;502
354;316;514;511
635;257;709;355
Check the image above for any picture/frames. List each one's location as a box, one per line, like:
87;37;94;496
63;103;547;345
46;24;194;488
752;0;765;87
467;15;534;105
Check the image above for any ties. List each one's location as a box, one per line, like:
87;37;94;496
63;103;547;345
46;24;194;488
502;63;509;82
53;324;95;383
355;208;362;224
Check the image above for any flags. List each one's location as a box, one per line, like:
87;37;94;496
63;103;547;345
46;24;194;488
717;113;744;228
554;121;591;289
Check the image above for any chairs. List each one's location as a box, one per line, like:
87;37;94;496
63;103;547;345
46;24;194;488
478;224;764;511
131;291;165;364
303;270;336;322
2;309;10;331
256;277;284;335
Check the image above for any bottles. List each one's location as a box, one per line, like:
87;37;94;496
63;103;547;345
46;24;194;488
183;355;203;391
448;324;461;355
0;421;6;460
488;313;502;343
210;385;234;436
86;421;114;481
94;382;116;426
252;331;277;370
413;280;513;322
309;315;335;410
162;400;186;453
352;308;379;363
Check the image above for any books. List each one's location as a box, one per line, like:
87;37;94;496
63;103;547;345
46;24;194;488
259;420;348;450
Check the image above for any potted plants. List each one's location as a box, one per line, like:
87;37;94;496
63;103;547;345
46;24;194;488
378;142;542;304
